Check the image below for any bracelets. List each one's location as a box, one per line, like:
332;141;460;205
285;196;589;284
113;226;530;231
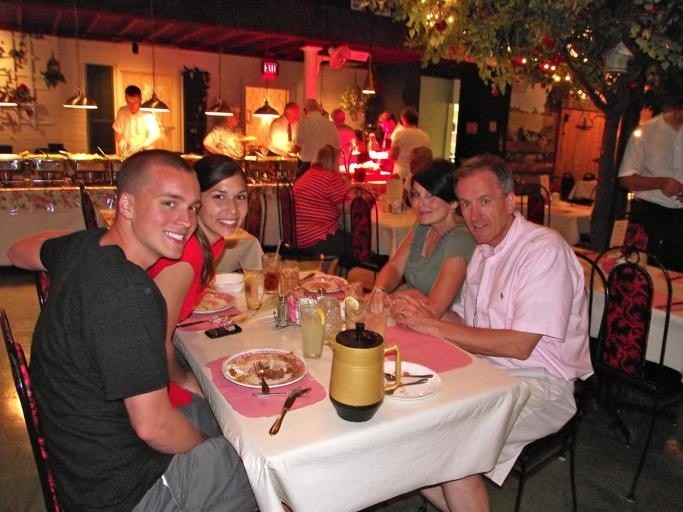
373;288;386;294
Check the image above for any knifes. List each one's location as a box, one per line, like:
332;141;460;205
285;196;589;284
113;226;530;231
176;314;237;328
268;386;301;437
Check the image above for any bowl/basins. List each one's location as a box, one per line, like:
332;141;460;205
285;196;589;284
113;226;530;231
212;272;245;294
603;251;650;273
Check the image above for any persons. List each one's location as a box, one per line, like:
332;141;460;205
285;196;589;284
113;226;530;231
9;149;259;511
148;153;250;408
370;157;480;320
617;73;683;274
112;85;166;161
420;155;595;511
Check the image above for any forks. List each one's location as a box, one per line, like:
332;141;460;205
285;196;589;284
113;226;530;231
253;361;272;396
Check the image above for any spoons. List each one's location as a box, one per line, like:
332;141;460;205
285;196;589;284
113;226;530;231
385;378;429;396
249;386;313;399
383;372;434;382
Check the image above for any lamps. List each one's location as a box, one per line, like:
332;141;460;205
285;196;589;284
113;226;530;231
203;1;237;118
60;1;97;111
135;1;172;114
252;0;281;118
0;89;19;109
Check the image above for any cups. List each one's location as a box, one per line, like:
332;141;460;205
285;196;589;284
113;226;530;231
552;192;560;207
241;250;392;358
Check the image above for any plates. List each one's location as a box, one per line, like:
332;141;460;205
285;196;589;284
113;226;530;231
383;360;443;402
191;289;240;315
220;346;309;390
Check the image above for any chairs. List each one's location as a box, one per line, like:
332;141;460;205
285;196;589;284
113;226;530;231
276;176;341;275
510;252;632;510
342;186;391;285
572;212;683;501
1;308;60;510
35;269;48;306
521;183;551;228
245;186;268;249
78;183;97;231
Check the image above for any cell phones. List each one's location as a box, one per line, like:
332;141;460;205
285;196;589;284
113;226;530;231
205;324;242;339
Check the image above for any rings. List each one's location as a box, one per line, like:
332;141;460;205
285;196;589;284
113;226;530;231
401;307;405;314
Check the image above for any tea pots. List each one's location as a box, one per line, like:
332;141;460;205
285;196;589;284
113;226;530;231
325;320;402;423
